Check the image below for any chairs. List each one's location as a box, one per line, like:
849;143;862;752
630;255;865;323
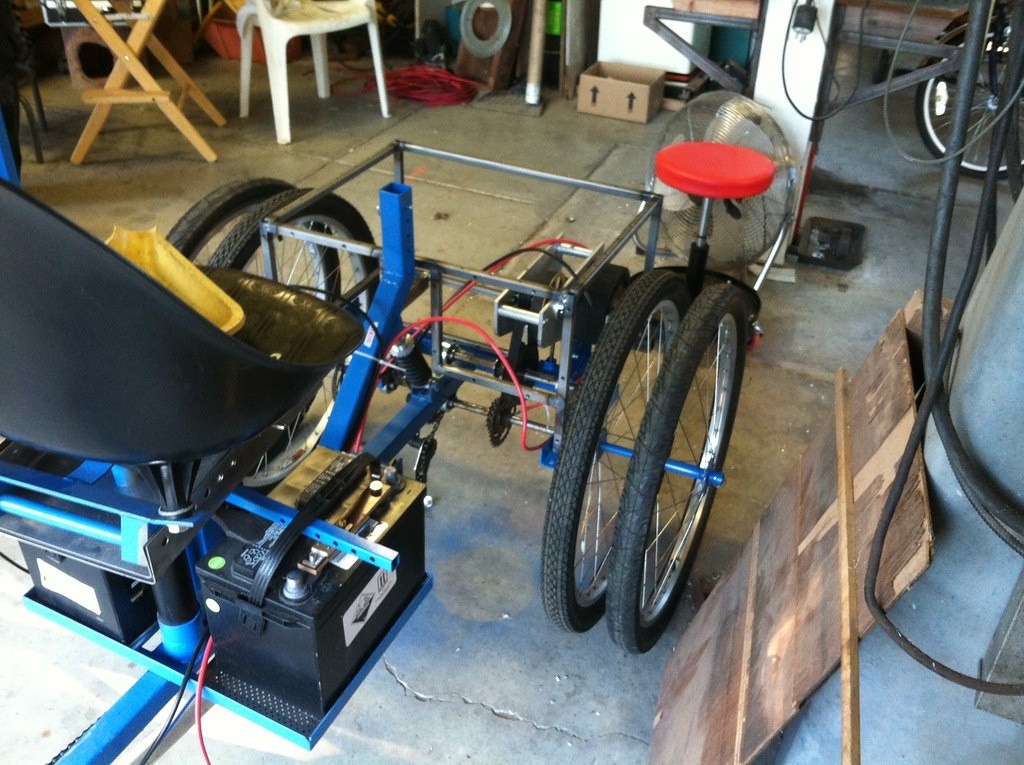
237;0;393;146
0;180;368;467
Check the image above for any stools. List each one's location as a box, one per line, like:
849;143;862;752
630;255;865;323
634;140;775;353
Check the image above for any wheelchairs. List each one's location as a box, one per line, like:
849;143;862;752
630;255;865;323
0;132;774;765
912;0;1024;182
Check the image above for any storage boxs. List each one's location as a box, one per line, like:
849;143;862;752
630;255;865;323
153;15;198;66
576;61;666;124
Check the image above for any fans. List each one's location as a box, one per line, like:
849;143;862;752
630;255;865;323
632;90;795;291
